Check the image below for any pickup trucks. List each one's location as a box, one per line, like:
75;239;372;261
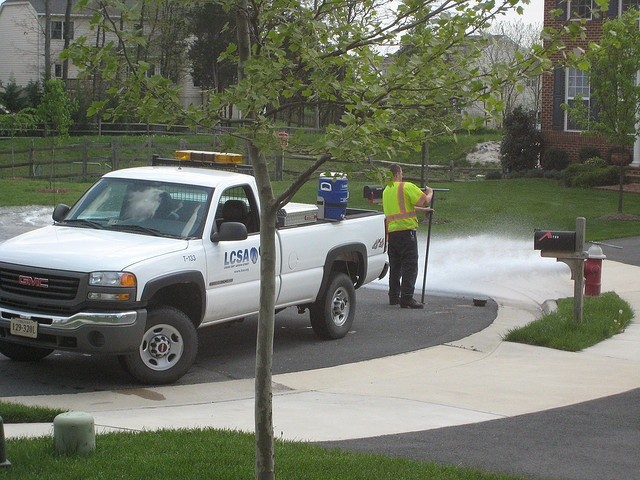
1;149;387;384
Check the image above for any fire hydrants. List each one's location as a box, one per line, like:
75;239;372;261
583;241;607;296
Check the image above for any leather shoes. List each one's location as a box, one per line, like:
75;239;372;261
400;298;424;309
389;296;401;305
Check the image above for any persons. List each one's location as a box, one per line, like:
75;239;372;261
382;165;435;309
